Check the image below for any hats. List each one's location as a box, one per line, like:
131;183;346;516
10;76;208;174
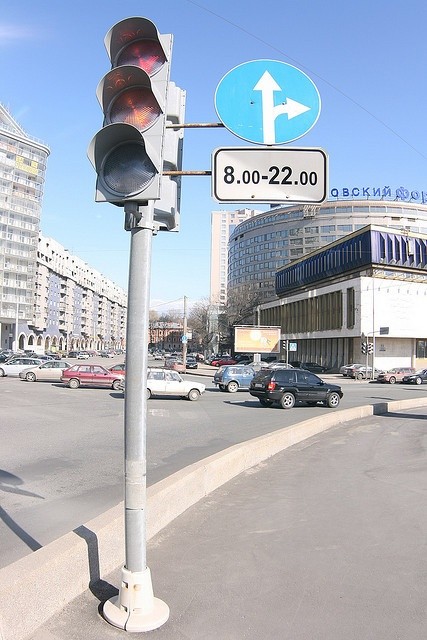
19;360;73;382
403;369;427;384
354;367;385;380
340;364;363;376
300;363;330;373
60;364;124;390
253;357;302;369
0;358;46;377
107;363;125;374
148;349;253;369
118;368;206;401
0;348;126;360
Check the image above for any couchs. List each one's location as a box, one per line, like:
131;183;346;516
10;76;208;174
369;344;372;353
84;15;169;197
282;340;286;351
362;342;367;354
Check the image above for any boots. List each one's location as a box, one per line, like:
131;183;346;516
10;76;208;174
212;365;258;393
377;367;417;384
250;369;344;409
